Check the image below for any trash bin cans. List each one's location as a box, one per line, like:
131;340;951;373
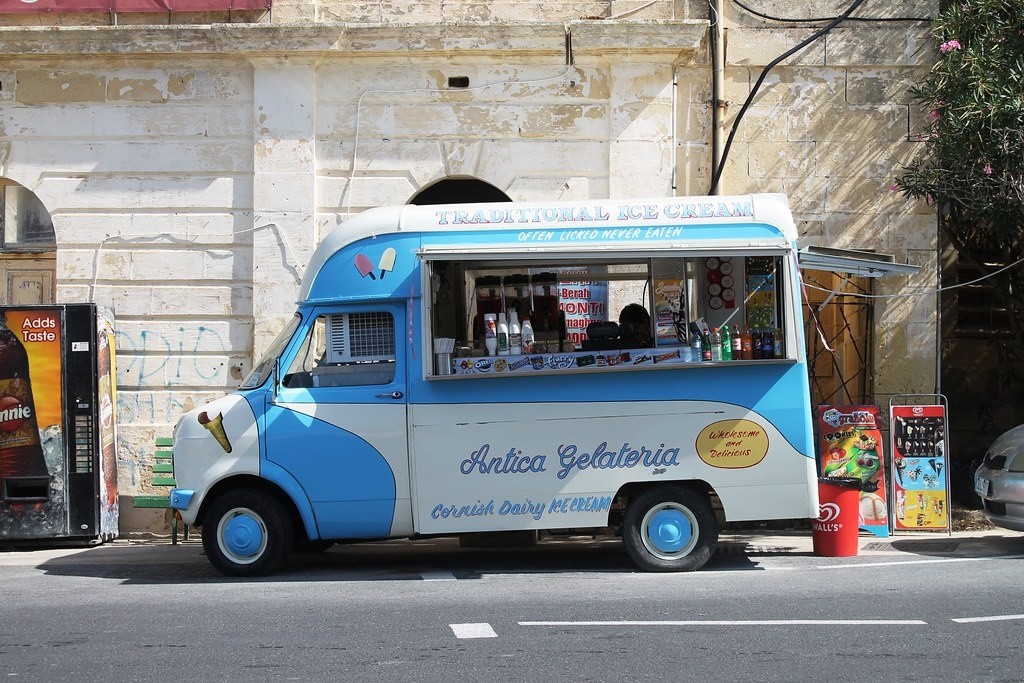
811;475;862;557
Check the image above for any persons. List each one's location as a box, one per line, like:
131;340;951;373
619;303;651;340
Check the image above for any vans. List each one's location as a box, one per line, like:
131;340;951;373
173;190;825;575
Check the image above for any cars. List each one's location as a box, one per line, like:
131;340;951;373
974;423;1024;534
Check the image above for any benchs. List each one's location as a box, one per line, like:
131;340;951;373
133;437;188;546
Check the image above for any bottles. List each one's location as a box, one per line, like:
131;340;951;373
731;324;743;361
752;324;763;360
509;312;522;355
691;331;702;363
741;325;753;360
721;326;733;361
709;327;722;362
496;313;510;357
521;317;534;354
702;328;712;362
562;340;575;352
534;341;547;353
774;328;783;359
483;313;497;357
762;324;774;359
547;340;560;354
506;308;516;324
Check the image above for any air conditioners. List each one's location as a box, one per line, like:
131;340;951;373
323;314;395;365
